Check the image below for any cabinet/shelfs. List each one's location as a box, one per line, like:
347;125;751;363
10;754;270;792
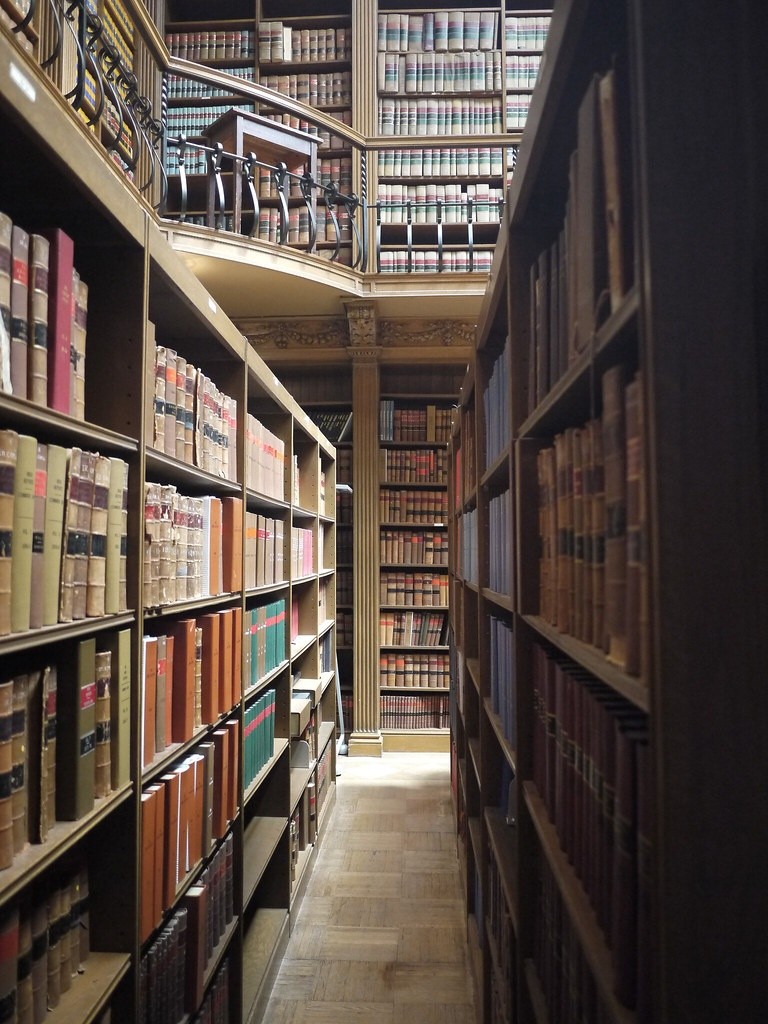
0;0;768;1024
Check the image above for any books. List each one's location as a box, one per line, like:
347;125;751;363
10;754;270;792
168;67;255;97
308;400;451;729
380;251;494;272
449;50;645;1024
378;149;504;176
259;71;352;150
167;104;254;174
377;52;502;93
377;98;502;135
506;55;543;88
506;147;519;190
259;158;352;267
505;17;551;51
175;214;233;232
165;21;352;63
0;210;131;1024
140;322;332;1023
379;184;503;223
506;94;533;127
378;13;499;51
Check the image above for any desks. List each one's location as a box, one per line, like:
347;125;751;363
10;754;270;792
201;107;326;252
